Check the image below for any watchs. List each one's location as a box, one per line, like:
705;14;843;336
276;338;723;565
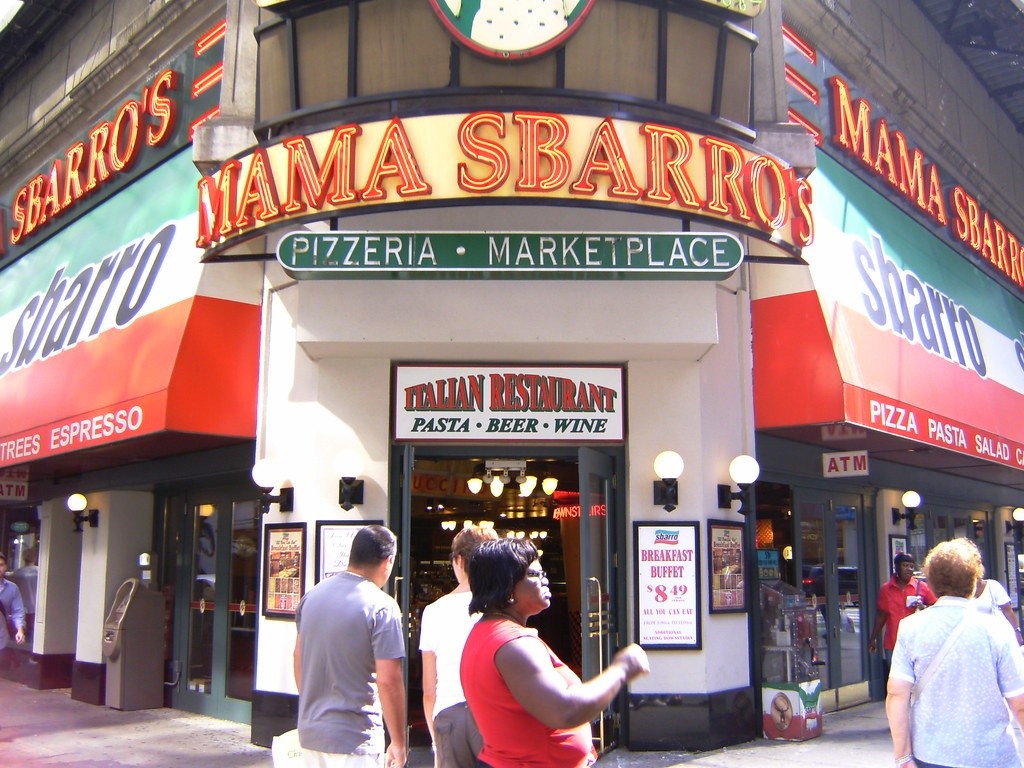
1015;627;1021;631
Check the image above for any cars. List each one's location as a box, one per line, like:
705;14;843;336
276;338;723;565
816;614;855;644
802;565;858;597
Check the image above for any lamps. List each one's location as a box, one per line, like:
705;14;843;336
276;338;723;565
66;495;99;533
718;455;760;516
482;469;494;483
466;473;483;494
653;451;683;513
892;492;920;531
498;469;510;483
516;469;527;483
253;457;295;514
1006;508;1024;540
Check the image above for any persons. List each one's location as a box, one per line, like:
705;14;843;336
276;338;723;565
418;525;498;768
293;525;408;767
458;537;651;767
867;537;1024;768
0;550;27;677
10;548;38;642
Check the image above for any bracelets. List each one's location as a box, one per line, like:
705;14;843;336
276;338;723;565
896;753;914;765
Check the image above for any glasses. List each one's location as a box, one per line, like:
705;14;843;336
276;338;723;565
525;570;546;579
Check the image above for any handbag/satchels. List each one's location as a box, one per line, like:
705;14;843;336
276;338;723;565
5;615;18;640
